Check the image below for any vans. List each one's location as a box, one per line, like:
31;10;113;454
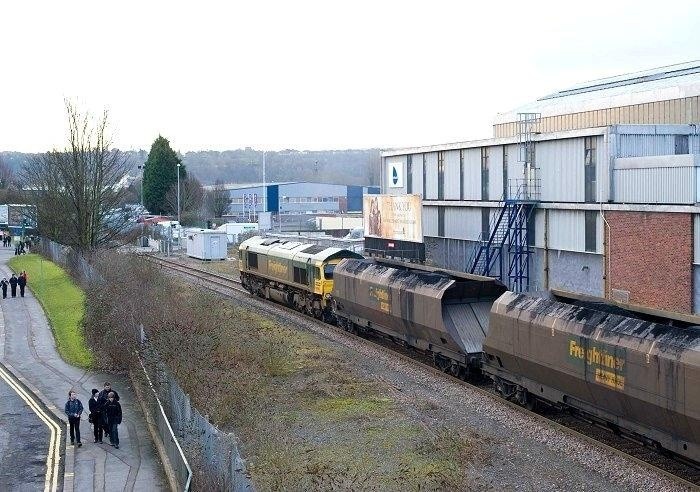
216;223;259;244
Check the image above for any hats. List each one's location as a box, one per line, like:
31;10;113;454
92;389;98;395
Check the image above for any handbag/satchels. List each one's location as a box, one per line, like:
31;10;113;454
88;413;95;423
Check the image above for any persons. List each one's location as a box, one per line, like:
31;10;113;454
0;271;30;300
0;226;40;256
64;382;123;449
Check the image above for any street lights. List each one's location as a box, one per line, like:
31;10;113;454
177;163;181;249
138;164;147;236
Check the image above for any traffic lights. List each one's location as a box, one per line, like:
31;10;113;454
244;193;248;208
254;194;257;208
248;194;253;206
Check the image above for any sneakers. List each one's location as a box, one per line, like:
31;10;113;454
71;439;75;444
77;440;82;446
93;432;119;449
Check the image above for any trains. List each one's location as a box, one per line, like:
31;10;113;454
239;234;699;466
